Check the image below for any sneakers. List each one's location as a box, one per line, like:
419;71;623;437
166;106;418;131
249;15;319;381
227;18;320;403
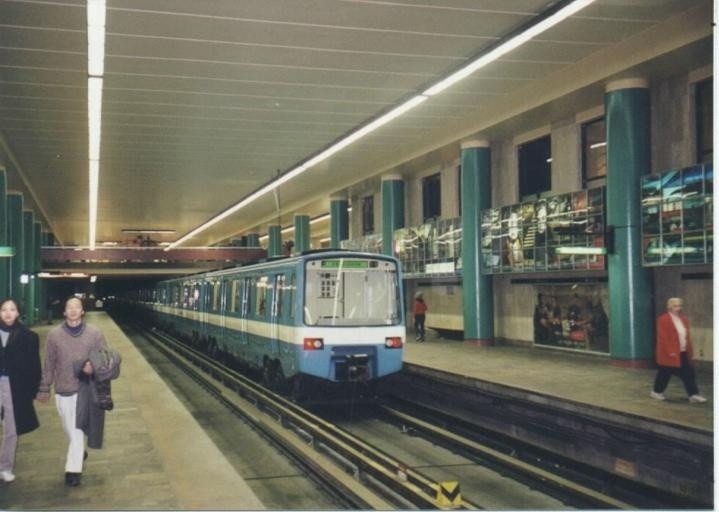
688;394;707;405
651;391;667;401
0;471;16;483
66;472;80;487
416;336;424;343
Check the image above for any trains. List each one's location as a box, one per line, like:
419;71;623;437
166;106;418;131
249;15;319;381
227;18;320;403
112;245;409;405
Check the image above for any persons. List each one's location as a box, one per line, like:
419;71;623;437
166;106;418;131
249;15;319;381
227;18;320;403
36;295;122;487
649;296;708;405
534;200;552;261
0;297;42;485
413;291;427;343
46;295;55;325
505;208;523;263
532;289;609;352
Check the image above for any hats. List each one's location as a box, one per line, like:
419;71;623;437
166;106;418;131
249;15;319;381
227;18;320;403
415;292;424;300
